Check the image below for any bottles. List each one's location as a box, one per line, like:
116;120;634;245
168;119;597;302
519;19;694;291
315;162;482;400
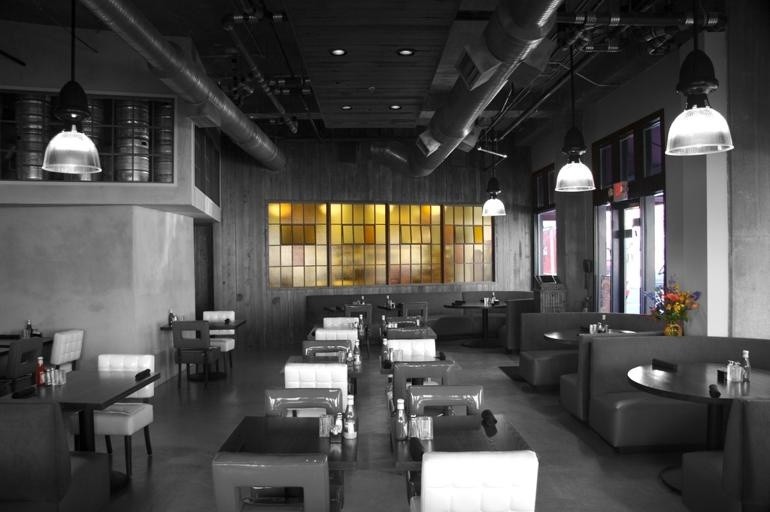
387;321;398;329
491;292;496;305
343;394;358;439
393;399;408;441
354;339;362;365
168;307;174;328
740;351;751;381
381;338;389;362
35;356;45;386
389;348;394;363
335;413;343;434
358;313;366;337
386;295;390;304
361;296;366;305
598;314;609;333
413;414;425;439
21;329;30;339
416;317;420;326
347;350;353;367
379;314;387;338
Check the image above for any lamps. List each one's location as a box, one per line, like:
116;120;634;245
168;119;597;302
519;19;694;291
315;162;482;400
482;133;506;217
42;0;103;173
554;48;596;193
665;0;734;156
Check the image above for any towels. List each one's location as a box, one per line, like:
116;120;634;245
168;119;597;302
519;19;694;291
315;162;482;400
225;319;230;324
409;437;424;461
455;300;466;305
709;384;721;397
135;369;151;381
652;358;678;372
12;387;34;397
482;410;497;426
384;360;392;369
436;351;446;361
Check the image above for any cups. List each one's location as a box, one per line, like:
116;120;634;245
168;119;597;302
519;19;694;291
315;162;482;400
26;320;32;338
420;416;433;441
494;300;500;305
727;360;735;381
731;362;744;382
394;350;403;362
45;367;59;385
321;415;334;427
319;418;330;438
337;351;346;363
589;323;598;335
407;417;420;439
484;298;490;306
55;370;66;385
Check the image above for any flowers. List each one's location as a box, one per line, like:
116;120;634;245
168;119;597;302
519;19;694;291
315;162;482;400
642;273;702;322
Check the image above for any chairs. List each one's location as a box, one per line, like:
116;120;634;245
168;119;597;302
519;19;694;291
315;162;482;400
407;385;485;416
387;339;437;361
93;354;155;478
392;361;456;403
213;453;329;512
314;328;358;356
0;397;110;511
173;321;221;385
386;314;422;327
410;451;539;512
323;317;359;328
0;338;43;398
265;388;342;416
303;340;352;357
50;329;84;370
203;311;236;374
285;361;347;412
345;305;372;357
404;302;428;322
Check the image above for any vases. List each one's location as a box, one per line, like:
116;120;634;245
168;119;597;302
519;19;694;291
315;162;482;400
664;322;682;336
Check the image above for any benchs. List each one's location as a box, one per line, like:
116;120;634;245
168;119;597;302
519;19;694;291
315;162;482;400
463;291;535;354
589;335;770;512
520;313;664;421
306;293;463;343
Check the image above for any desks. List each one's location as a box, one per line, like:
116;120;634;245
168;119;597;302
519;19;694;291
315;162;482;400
443;301;508;349
545;329;637;348
396;413;532;501
213;414;360;512
378;305;404;317
160;320;246;381
627;362;770;496
381;361;424;385
387;327;437;339
280;356;354;375
323;305;347;313
0;337;54;353
0;369;162;494
